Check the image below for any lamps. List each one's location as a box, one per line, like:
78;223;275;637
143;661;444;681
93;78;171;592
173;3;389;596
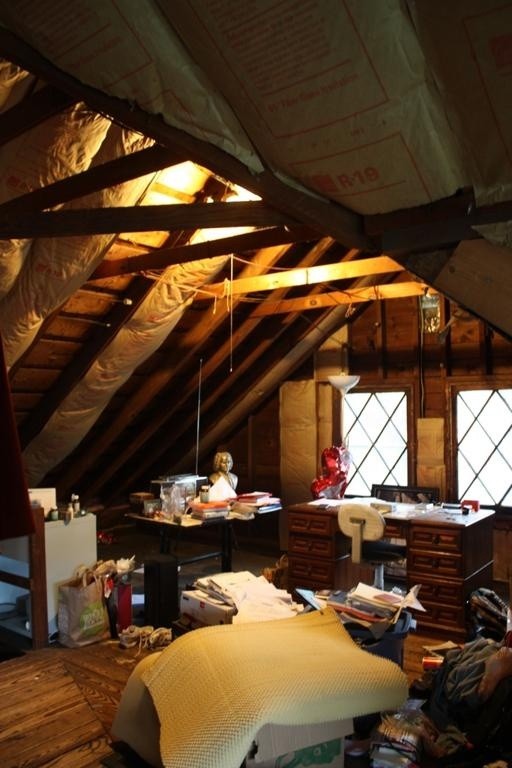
326;290;361;398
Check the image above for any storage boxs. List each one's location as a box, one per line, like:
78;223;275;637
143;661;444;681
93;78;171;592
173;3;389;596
305;602;412;675
243;718;355;767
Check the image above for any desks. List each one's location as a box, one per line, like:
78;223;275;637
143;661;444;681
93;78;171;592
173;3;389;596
122;509;283;629
286;496;498;646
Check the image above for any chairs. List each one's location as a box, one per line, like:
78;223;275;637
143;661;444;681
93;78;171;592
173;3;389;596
336;503;408;589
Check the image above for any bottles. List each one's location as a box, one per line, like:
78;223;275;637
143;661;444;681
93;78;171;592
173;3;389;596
199;485;210;503
49;496;80;521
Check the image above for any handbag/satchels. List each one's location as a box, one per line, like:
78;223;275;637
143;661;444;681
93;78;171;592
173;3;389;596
57;565;113;648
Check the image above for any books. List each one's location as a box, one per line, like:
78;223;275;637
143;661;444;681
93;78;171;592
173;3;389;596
294;581;407;628
188;490;284;524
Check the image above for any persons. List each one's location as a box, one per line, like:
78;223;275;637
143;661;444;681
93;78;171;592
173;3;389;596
207;450;239;491
